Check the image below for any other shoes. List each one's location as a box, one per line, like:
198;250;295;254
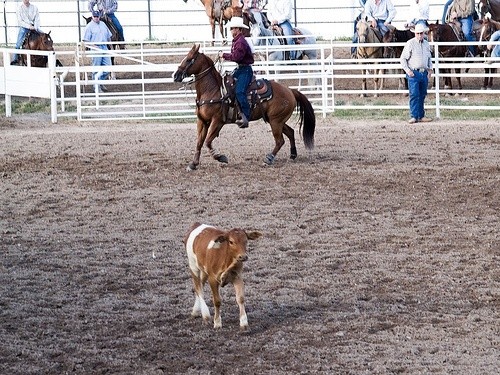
101;85;108;91
236;120;248;128
420;118;432;121
409;117;416;123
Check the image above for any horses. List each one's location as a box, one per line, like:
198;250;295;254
82;15;118;65
17;29;55;68
248;21;318;91
352;16;403;98
380;17;500;98
184;0;250;46
171;45;317;171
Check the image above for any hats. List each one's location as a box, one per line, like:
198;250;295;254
410;24;430;33
93;11;99;17
226;16;249;30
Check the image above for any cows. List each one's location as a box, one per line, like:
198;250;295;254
183;223;263;334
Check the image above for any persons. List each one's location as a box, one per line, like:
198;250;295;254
87;0;125;49
449;0;475;57
364;0;394;36
400;24;432;123
267;0;296;59
218;17;254;126
243;0;268;24
487;30;500;57
82;11;115;92
11;0;43;65
404;0;429;28
